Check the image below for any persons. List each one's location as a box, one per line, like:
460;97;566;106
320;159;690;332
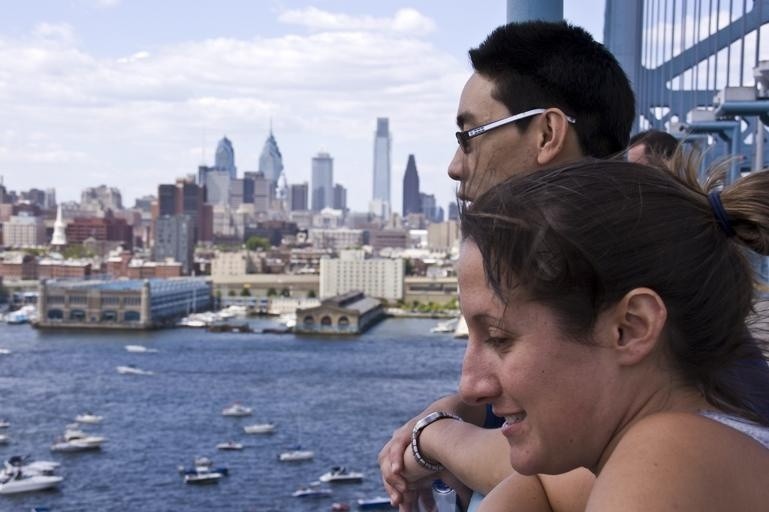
629;131;684;171
455;144;769;512
378;20;769;512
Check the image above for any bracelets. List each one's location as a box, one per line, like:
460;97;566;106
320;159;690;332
410;409;463;472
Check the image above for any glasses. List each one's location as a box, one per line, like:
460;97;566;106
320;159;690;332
455;106;579;155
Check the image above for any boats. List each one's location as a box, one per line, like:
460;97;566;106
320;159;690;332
178;301;245;329
431;319;456;333
2;303;37;325
176;402;400;511
0;345;163;498
